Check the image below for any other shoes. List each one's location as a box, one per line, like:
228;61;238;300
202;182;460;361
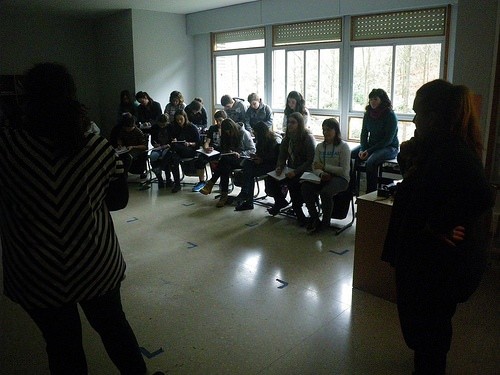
216;197;227;207
158;178;164;188
198;184;212;196
226;197;242;205
234;201;254;210
270;200;289;215
296;209;305;226
172;184;181;193
165;179;173;187
192;182;206;192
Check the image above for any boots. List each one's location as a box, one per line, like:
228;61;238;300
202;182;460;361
311;210;332;233
305;204;320;229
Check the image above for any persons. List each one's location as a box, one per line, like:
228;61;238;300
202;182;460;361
164;91;184;123
1;64;149;375
301;118;350;235
267;111;315;223
191;111;227;191
232;121;279;210
244;93;272;136
221;95;245;123
118;90;139;120
127;91;162;145
165;109;199;193
184;97;207;133
150;112;172;187
199;118;256;207
109;112;147;174
351;89;399;196
284;91;307;135
380;79;485;375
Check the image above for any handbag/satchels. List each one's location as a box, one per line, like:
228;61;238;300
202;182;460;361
234;171;244;186
266;180;288;198
330;198;350;219
181;161;200;175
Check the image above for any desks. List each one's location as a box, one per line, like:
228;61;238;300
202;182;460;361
353;180;420;305
358;145;404;187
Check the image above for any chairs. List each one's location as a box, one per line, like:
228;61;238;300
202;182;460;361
123;126;357;238
